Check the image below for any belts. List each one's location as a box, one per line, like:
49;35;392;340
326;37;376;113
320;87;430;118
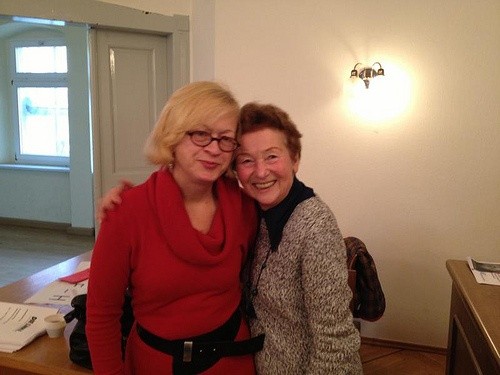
135;307;266;375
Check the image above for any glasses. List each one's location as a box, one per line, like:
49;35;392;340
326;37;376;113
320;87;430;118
185;130;242;153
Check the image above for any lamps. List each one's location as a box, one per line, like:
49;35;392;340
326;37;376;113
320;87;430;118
343;61;393;91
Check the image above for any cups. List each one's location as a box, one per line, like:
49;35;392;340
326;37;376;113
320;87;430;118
44;314;65;338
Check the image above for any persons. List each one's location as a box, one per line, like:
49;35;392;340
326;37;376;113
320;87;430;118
85;81;259;375
98;98;365;375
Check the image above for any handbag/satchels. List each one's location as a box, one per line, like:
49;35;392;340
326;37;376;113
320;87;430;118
63;294;135;370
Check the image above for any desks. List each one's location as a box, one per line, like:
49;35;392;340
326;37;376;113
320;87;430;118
1;248;96;374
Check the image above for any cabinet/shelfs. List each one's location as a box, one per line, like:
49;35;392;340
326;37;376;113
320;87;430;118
446;259;500;375
